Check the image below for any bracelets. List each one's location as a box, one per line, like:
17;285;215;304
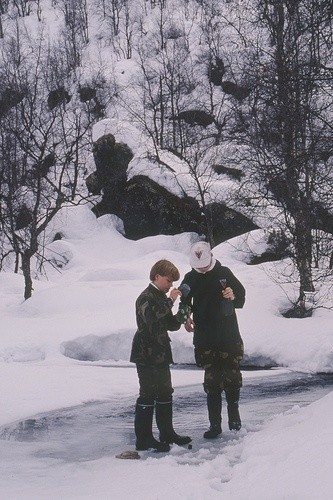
170;297;174;303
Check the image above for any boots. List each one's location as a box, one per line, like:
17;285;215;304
227;404;241;432
203;412;222;439
155;401;192;445
134;404;170;452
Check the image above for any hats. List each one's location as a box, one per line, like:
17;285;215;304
190;241;210;268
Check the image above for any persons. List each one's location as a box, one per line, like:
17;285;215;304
131;259;193;453
179;242;246;439
